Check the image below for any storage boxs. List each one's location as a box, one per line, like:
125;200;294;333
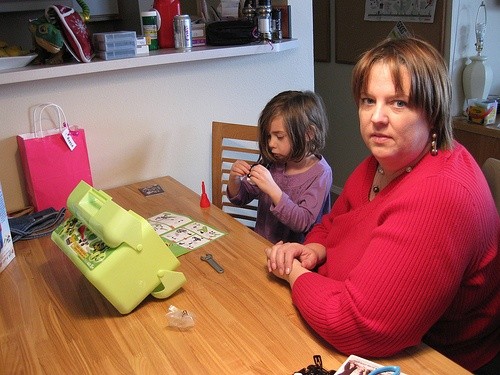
93;31;150;61
468;98;497;125
51;180;185;315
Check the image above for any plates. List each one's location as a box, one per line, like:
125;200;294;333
0;51;38;69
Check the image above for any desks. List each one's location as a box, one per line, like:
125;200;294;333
0;177;477;375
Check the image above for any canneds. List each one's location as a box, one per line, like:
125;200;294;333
173;15;192;49
141;11;158;50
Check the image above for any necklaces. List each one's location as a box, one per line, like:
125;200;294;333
373;166;413;193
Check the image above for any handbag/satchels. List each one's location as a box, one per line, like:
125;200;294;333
16;104;94;212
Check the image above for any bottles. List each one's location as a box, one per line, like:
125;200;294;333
242;0;256;23
257;0;272;44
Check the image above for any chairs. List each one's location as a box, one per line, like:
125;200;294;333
212;120;261;233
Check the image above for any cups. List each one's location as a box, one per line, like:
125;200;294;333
140;11;159;50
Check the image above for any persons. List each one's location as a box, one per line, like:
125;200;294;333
264;36;500;375
226;90;331;245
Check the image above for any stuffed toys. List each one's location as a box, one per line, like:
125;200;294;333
27;18;65;64
